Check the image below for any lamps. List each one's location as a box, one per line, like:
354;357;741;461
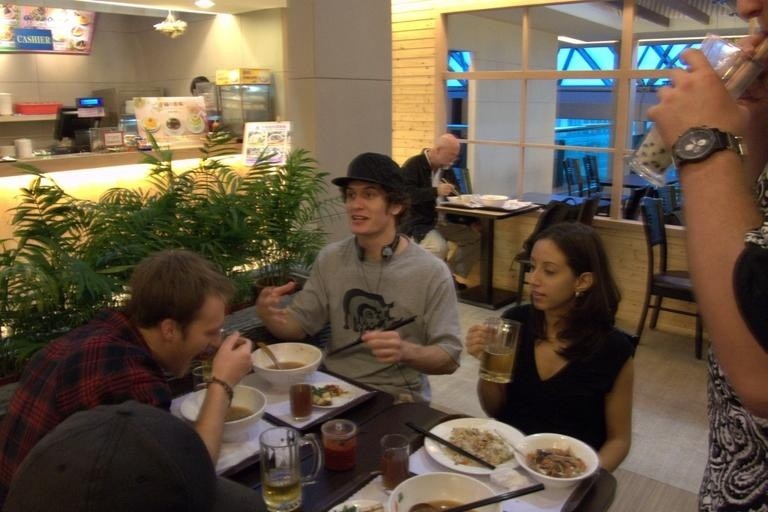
153;10;188;38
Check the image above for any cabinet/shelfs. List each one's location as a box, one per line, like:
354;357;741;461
218;84;272;138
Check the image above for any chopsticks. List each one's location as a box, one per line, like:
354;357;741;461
326;313;417;357
261;410;297;428
439;177;461;200
403;420;494;469
442;481;545;511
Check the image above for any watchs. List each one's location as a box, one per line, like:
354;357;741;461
671;124;748;169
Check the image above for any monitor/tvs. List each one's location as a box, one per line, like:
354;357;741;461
53;105;102;154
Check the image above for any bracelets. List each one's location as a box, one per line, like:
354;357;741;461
206;377;234;408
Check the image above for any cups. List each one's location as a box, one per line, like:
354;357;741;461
257;425;321;512
287;372;313;424
192;365;211;391
476;317;522;385
378;433;410;492
627;30;765;190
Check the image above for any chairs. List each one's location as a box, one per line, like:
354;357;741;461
634;197;703;360
563;154;683;226
514;195;600;306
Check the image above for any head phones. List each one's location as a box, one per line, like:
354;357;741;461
352;234;401;260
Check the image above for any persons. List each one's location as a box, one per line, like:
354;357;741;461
465;218;642;476
189;76;210;96
643;0;768;512
0;249;254;511
255;149;465;405
398;133;483;292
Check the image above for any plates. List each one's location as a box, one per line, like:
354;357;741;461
141;118;160;133
269;133;283;142
0;92;13;115
186;117;205;133
0;137;33;159
421;418;526;477
311;381;354;408
326;498;383;512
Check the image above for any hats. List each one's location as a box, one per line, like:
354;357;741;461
333;152;405;192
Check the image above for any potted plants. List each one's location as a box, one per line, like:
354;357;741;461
0;147;345;385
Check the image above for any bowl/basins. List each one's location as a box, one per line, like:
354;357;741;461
445;191;507;209
514;432;600;491
179;383;267;442
249;341;323;391
165;117;181;133
385;474;501;511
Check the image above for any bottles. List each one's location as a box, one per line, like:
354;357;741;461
321;418;359;470
